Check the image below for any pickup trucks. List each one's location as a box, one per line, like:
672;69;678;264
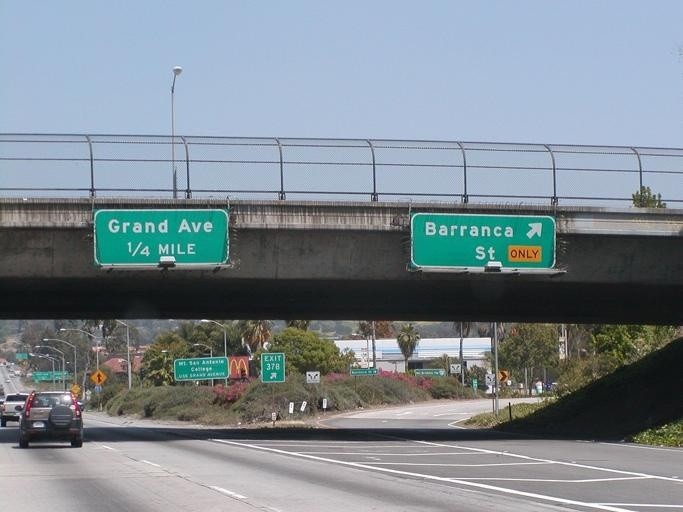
1;392;29;427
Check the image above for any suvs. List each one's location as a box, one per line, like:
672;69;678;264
14;390;85;448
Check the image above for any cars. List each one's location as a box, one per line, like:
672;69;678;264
0;399;3;418
0;360;31;385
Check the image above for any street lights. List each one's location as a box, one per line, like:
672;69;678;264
199;319;228;387
42;336;77;384
193;343;213;387
27;351;55;384
169;65;182;197
352;332;370;368
58;327;99;372
160;348;167;365
115;319;131;391
34;344;66;389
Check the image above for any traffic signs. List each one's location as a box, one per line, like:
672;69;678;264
175;358;230;381
413;368;446;376
93;208;230;268
351;369;378;376
15;352;27;359
412;215;555;272
32;371;68;381
262;353;286;383
